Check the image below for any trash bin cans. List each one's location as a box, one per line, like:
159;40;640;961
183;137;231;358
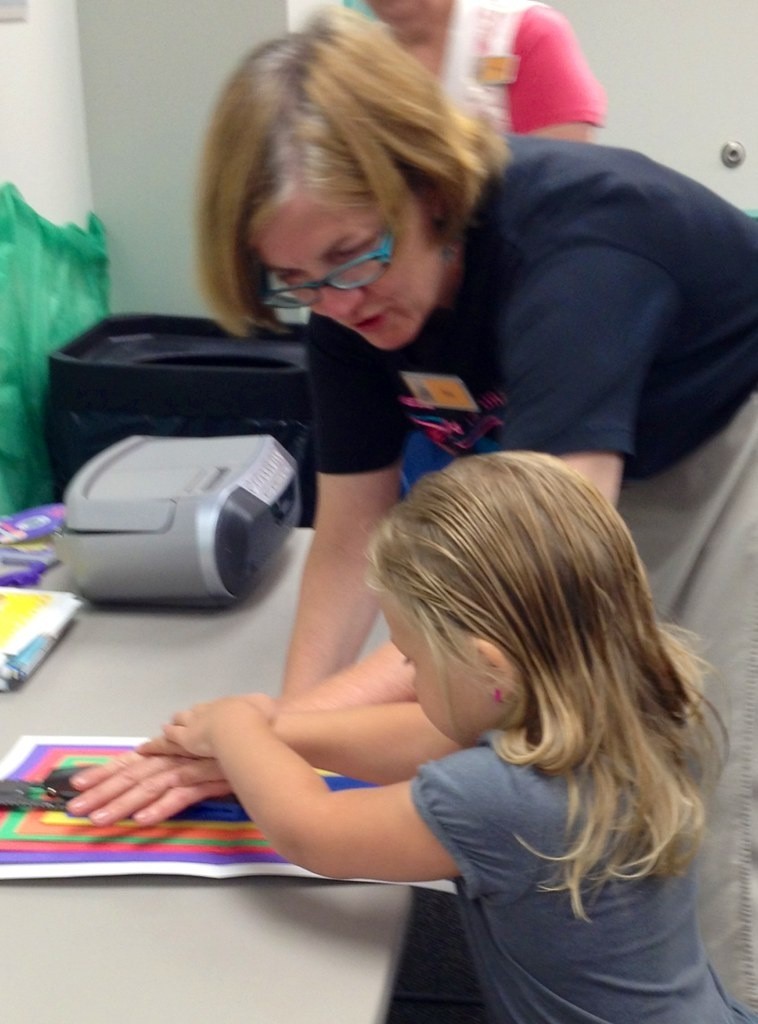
38;305;321;534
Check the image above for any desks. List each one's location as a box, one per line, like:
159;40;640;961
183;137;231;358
0;522;418;1024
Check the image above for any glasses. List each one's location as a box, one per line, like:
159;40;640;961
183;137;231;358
257;225;397;310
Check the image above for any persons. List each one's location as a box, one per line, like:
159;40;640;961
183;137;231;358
356;0;603;144
64;30;758;1023
133;445;751;1024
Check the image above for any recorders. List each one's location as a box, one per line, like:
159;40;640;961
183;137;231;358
52;433;303;607
